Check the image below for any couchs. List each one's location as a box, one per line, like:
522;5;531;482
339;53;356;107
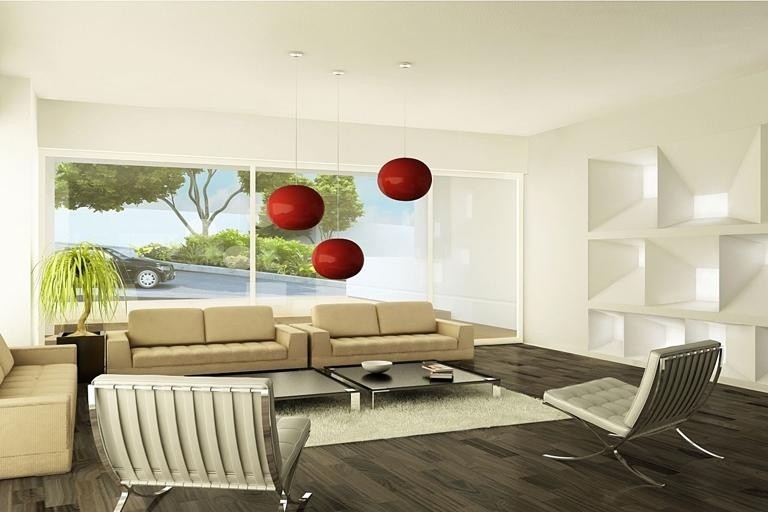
101;304;311;375
0;334;81;478
289;300;477;364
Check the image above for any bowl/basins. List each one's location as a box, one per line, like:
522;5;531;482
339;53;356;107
361;360;392;374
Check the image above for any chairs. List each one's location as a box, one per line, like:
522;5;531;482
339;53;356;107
539;339;729;488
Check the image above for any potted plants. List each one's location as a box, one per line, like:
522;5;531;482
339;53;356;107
34;239;128;380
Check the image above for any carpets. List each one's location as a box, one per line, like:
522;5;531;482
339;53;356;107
268;384;573;448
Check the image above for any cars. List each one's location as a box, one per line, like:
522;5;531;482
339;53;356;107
91;247;176;288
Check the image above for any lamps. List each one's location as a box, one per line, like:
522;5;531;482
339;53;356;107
376;61;433;199
268;50;365;281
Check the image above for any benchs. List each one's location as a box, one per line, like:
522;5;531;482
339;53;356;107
85;371;314;512
